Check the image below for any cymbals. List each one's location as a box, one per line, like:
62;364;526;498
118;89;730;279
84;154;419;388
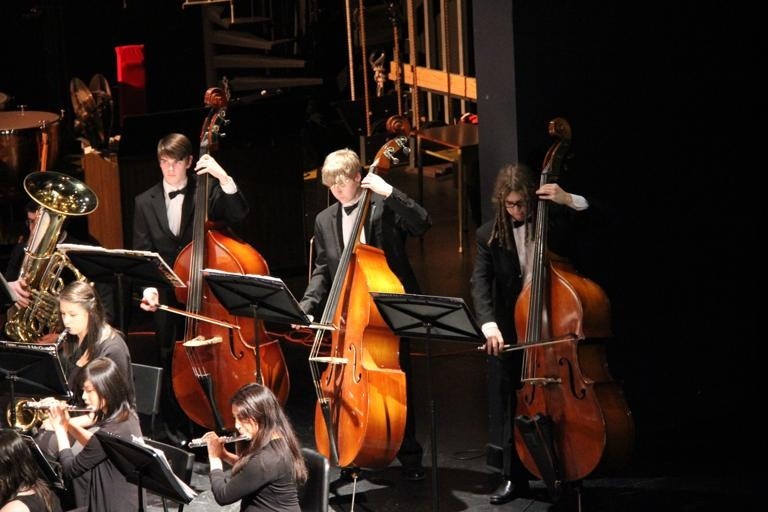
69;74;112;144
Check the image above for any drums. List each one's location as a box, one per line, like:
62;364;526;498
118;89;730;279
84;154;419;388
2;110;62;201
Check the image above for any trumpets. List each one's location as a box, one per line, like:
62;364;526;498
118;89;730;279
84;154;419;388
5;397;86;436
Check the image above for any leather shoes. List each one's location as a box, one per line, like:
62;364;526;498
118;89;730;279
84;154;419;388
400;463;426;481
490;477;530;505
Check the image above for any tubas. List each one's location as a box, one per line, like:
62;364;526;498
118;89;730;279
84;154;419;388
5;169;97;349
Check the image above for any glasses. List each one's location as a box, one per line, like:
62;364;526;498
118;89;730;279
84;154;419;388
504;200;525;208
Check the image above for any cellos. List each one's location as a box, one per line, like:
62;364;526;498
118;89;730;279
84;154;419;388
171;85;291;436
309;116;407;470
513;117;631;488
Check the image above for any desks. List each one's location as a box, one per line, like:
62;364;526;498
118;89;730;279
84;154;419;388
410;123;480;253
0;111;60;168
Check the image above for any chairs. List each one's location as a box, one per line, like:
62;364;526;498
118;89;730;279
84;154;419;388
67;363;331;512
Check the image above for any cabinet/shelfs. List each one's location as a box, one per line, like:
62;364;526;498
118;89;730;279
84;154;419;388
83;146;124;249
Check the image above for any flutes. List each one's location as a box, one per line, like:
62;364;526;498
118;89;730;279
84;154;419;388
188;434;253;449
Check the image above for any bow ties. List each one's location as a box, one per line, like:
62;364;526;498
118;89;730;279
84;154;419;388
344;202;358;215
513;216;532;227
169;186;188;199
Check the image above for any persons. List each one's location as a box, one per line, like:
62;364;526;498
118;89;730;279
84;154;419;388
41;358;147;512
132;132;250;433
1;274;30;310
2;201;114;325
202;382;308;512
1;429;61;512
37;281;136;461
469;164;591;506
299;147;434;484
